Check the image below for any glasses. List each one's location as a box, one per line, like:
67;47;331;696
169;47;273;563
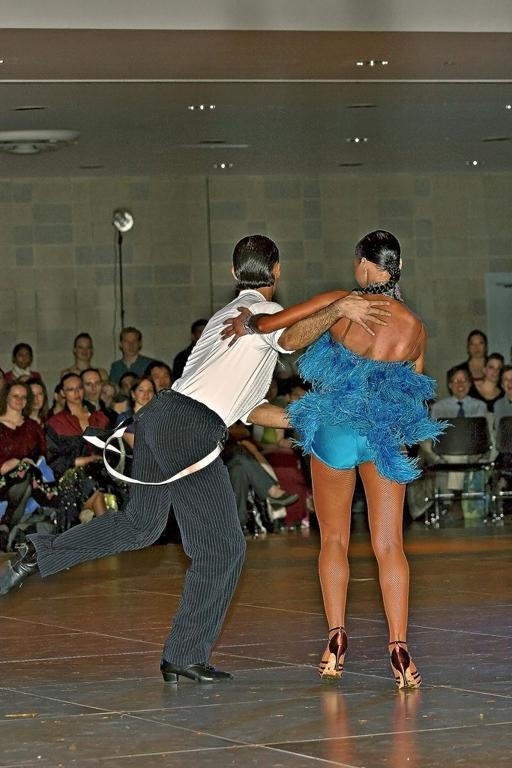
31;391;46;401
446;375;469;385
5;393;27;401
84;379;100;390
61;384;86;394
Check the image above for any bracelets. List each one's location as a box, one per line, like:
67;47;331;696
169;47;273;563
243;314;249;334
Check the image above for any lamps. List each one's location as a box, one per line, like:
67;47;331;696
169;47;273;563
110;204;135;231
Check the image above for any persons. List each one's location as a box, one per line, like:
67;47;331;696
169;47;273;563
47;373;108;517
121;371;138;399
0;235;392;685
466;354;507;414
4;343;45;396
449;329;490;377
110;326;167;385
173;317;211;378
119;380;155;451
48;385;66;419
433;369;486;519
217;229;456;693
99;381;117;412
248;376;314;459
494;365;512;462
58;331;108;382
3;341;39;380
148;362;173;395
0;381;48;532
219;422;299;537
27;380;49;435
81;367;108;414
235;439;287;534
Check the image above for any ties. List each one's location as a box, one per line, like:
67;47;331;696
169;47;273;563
455;398;465;419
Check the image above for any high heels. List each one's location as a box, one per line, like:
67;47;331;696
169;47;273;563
158;657;238;692
1;534;42;603
243;514;268;539
314;623;350;682
262;486;297;509
385;637;425;695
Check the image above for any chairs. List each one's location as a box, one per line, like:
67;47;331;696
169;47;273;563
491;416;512;524
426;414;497;529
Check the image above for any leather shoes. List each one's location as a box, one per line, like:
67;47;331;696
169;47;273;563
414;501;451;526
